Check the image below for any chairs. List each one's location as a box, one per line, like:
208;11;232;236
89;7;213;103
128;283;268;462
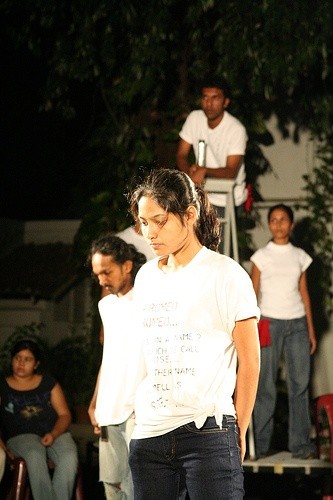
12;438;83;500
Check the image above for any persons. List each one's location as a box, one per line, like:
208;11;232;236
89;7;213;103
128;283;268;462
177;75;247;256
88;235;146;500
250;203;319;460
125;168;261;500
0;340;78;500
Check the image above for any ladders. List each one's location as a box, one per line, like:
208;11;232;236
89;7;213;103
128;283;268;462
201;180;258;461
